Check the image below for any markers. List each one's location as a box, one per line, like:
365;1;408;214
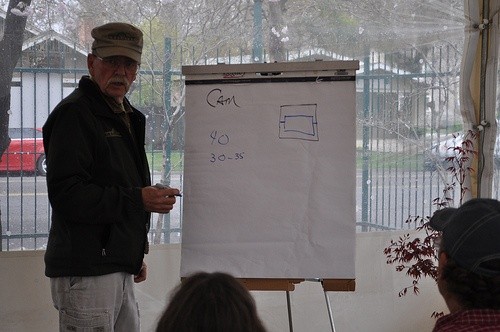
156;183;182;198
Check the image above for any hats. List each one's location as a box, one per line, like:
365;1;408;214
430;198;500;278
90;23;143;63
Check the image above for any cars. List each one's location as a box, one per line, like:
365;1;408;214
422;116;500;172
0;127;48;177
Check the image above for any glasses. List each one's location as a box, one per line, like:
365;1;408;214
95;55;140;74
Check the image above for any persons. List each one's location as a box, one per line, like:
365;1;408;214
42;22;179;332
429;198;500;332
155;270;270;332
